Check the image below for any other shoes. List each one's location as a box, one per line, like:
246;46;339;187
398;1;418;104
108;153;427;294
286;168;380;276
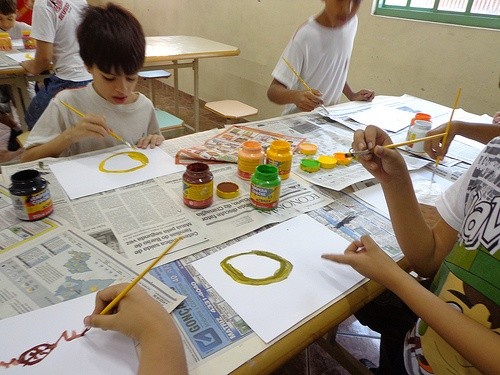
359;358;377;369
8;128;23;151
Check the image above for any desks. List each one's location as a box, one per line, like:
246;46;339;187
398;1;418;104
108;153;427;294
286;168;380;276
0;97;494;375
0;36;241;134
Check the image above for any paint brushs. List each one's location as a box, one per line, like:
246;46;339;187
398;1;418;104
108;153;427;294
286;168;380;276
345;133;447;157
432;88;462;179
80;236;181;336
282;57;329;114
60;100;131;148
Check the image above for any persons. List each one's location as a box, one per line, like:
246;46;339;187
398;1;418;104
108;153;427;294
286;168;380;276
266;1;378;119
83;277;190;375
320;122;500;375
15;2;165;170
0;0;45;152
19;0;98;141
423;68;500;174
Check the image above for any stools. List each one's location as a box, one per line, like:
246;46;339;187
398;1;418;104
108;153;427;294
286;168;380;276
156;109;184;133
205;100;259;124
16;131;30;146
137;69;172;106
